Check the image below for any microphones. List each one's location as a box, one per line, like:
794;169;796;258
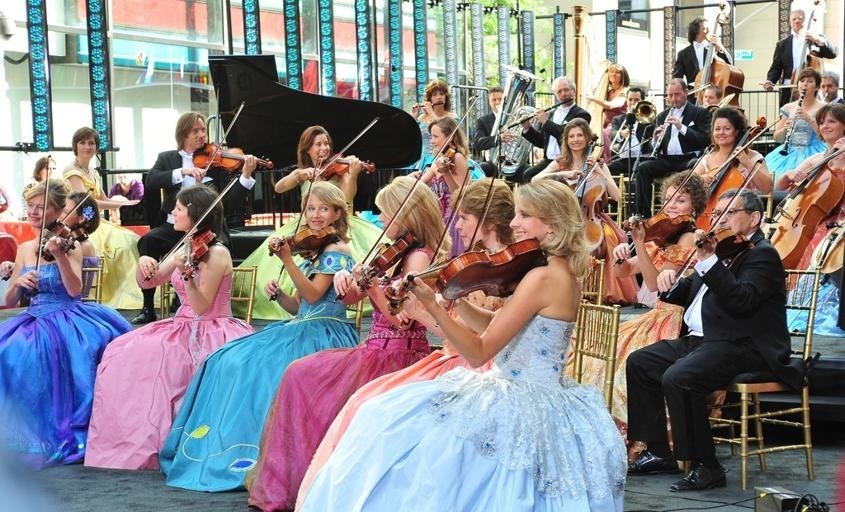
626;113;635;130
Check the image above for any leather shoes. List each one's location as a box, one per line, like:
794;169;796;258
670;460;726;492
626;449;678;475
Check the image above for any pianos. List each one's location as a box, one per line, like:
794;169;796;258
209;55;422;227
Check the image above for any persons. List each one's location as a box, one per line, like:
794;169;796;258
415;62;724;216
2;176;134;467
669;16;731;97
296;180;629;511
25;110;374;214
292;178;518;512
703;73;842;336
244;176;447;512
629;189;793;491
84;183;254;473
763;6;836;103
158;182;366;495
566;168;729;461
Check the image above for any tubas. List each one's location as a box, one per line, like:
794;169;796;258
484;63;542;175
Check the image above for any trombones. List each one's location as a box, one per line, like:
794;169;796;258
609;90;657;154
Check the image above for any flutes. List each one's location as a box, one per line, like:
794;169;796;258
412;100;444;109
507;98;573;128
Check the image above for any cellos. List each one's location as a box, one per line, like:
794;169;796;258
695;0;845;292
561;134;608;252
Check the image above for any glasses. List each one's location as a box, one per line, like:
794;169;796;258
711;209;749;217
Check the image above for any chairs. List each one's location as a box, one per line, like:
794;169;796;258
78;171;825;492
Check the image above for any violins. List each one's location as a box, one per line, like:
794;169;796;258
319;152;377;180
192;141;273;174
437;148;457;174
71;225;89;242
269;226;339;252
383;238;550;316
358;233;418;292
621;214;695;249
34;221;77;262
178;229;217;282
